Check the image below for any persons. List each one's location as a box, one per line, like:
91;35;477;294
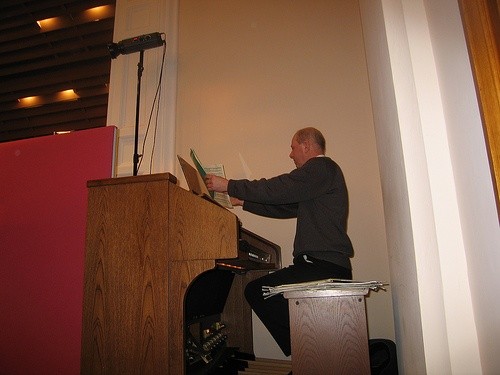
204;128;355;375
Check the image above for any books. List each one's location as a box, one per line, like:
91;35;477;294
189;148;234;210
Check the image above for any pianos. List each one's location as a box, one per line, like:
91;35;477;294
80;171;283;375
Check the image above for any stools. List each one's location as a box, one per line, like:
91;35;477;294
283;288;371;375
